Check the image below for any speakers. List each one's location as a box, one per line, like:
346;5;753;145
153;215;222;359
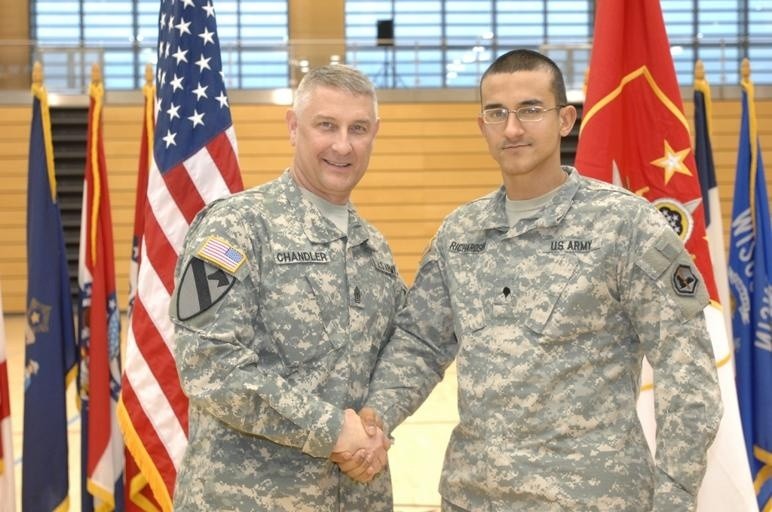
378;21;393;46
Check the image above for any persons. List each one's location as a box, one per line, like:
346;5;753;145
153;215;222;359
332;49;722;511
169;64;412;511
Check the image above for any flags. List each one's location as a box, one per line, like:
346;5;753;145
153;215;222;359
117;1;244;511
79;96;125;512
727;76;772;510
23;84;82;512
692;82;730;308
574;0;759;510
2;311;17;511
126;82;157;320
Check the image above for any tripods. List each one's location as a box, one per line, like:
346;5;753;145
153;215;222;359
372;46;407;88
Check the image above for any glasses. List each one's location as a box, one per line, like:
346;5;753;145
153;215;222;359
483;105;563;123
